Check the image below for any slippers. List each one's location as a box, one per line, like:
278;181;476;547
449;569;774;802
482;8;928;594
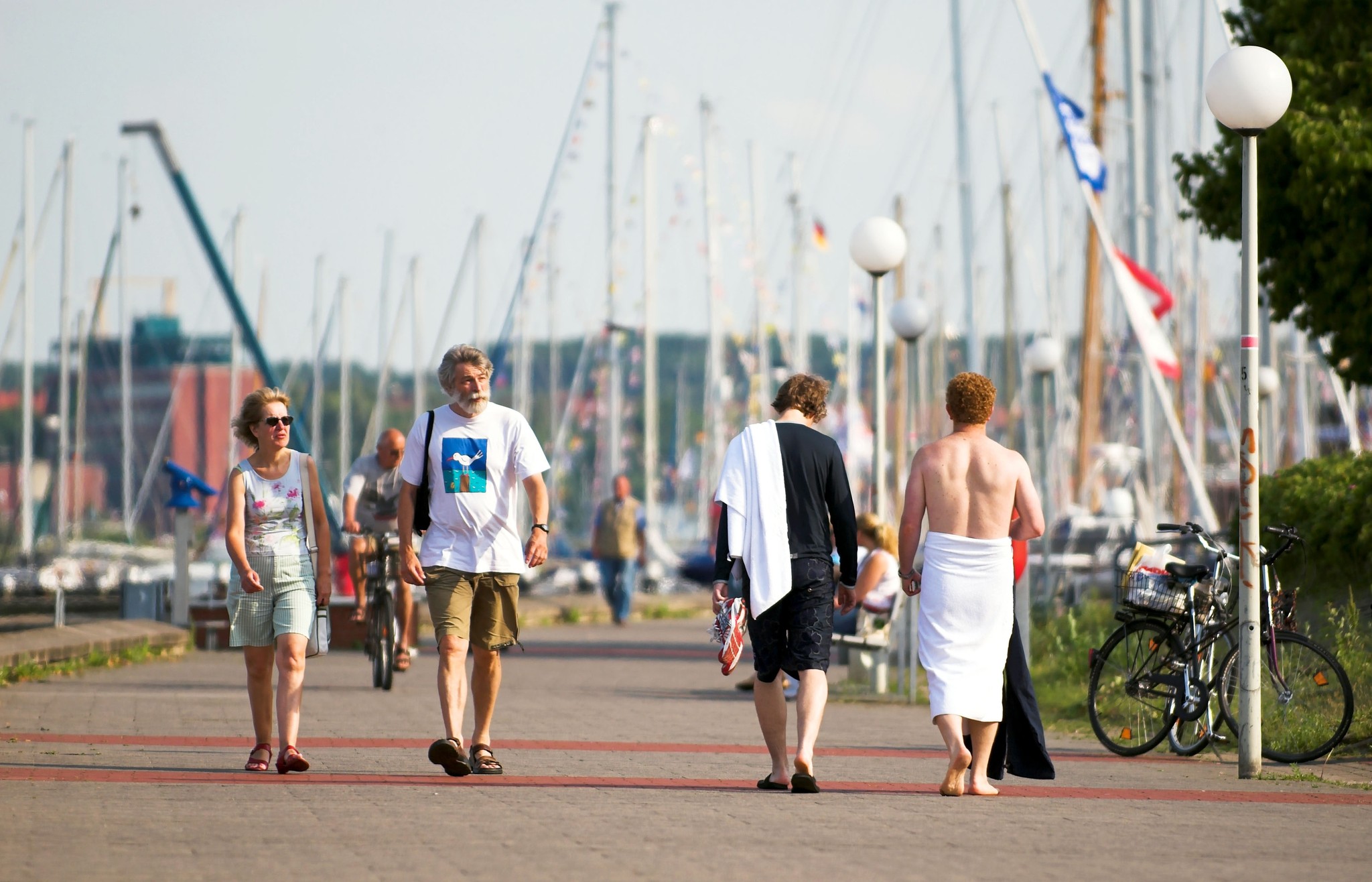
791;773;820;793
757;773;788;790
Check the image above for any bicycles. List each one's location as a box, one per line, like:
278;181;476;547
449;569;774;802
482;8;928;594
1085;520;1356;767
340;524;422;691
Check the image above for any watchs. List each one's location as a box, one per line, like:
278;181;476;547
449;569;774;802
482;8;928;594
531;524;549;534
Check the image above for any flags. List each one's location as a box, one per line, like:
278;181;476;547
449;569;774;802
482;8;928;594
1043;70;1181;384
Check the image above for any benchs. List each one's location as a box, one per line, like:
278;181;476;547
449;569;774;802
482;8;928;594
831;590;904;694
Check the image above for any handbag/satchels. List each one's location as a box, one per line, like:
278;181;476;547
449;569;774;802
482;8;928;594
413;410;435;537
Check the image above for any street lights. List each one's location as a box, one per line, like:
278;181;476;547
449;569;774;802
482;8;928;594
845;214;932;531
1025;332;1066;621
1203;44;1294;781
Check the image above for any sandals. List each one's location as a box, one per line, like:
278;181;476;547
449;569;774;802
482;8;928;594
245;743;271;771
349;601;367;622
393;644;411;672
468;744;503;774
275;745;309;773
428;737;471;777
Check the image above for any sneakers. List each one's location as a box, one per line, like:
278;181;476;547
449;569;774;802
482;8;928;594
706;597;746;664
721;607;748;676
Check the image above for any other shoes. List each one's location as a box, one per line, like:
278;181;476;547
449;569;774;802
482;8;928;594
735;671;786;690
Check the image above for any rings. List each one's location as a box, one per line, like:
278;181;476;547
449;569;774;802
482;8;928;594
404;578;407;581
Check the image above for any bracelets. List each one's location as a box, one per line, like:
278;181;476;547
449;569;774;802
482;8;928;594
898;567;915;579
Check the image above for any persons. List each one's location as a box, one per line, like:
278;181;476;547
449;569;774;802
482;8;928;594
592;473;648;628
225;386;331;775
342;428;425;673
711;374;858;793
397;343;551;778
733;511;901;701
898;373;1046;796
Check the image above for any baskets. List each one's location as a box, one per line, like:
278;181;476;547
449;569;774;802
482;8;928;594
1114;567;1213;625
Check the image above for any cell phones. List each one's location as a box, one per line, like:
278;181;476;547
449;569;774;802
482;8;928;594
909;580;919;592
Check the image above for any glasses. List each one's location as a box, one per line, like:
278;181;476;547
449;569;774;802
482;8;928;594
258;416;294;427
389;449;404;455
